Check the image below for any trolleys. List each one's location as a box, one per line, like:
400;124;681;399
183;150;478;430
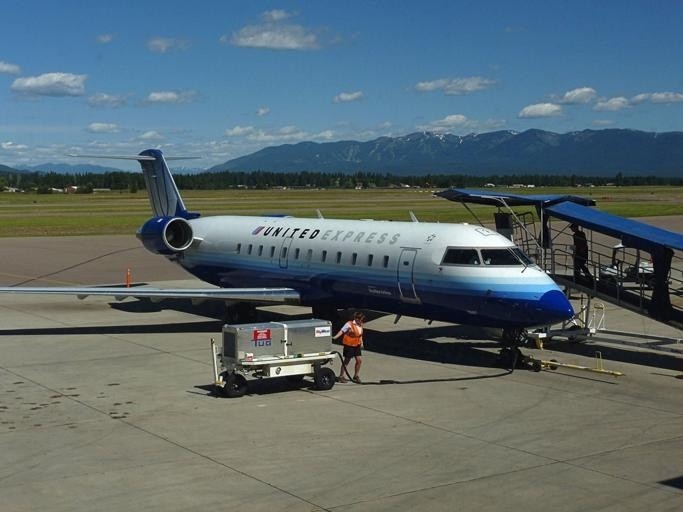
210;339;335;397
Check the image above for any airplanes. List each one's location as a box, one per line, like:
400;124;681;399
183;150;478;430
1;149;574;367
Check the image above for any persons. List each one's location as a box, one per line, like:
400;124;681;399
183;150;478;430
331;310;365;384
566;222;593;284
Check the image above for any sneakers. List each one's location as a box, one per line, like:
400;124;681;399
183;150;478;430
337;375;361;383
